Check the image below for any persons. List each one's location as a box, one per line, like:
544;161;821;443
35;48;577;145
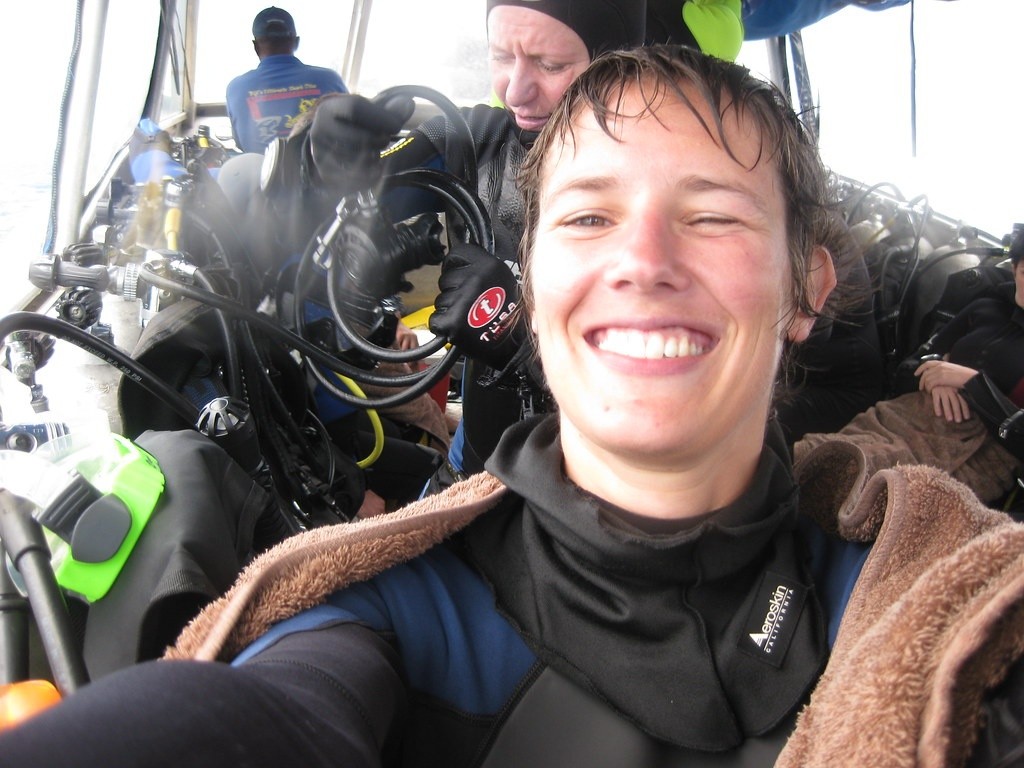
1;46;1024;768
117;0;688;518
228;7;347;155
806;206;871;346
897;224;1023;466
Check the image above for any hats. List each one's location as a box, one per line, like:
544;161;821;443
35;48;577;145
486;0;647;151
252;6;297;37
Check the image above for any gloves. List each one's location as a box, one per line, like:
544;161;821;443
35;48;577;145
309;93;416;193
429;242;531;373
